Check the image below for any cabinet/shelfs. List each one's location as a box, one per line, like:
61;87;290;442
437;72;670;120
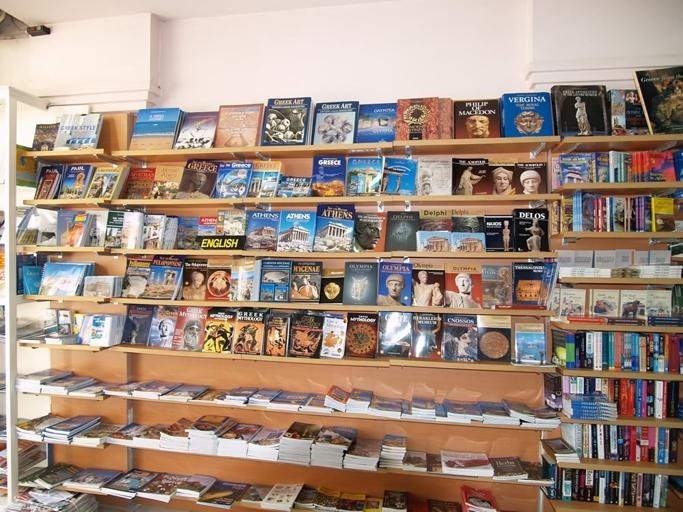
1;86;45;512
21;112;683;512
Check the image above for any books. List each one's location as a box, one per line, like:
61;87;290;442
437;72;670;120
398;96;453;139
545;462;671;510
551;285;683;329
605;88;647;135
544;374;683;420
173;110;217;151
555;326;683;374
501;88;554;137
130;107;182;151
32;123;57;151
551;84;610;137
54;113;105;149
218;98;263;149
556;247;683;279
550;193;683;233
550;149;683;182
354;101;398;143
260;96;316;145
633;63;683;134
456;101;500;135
312;99;362;142
542;424;682;467
